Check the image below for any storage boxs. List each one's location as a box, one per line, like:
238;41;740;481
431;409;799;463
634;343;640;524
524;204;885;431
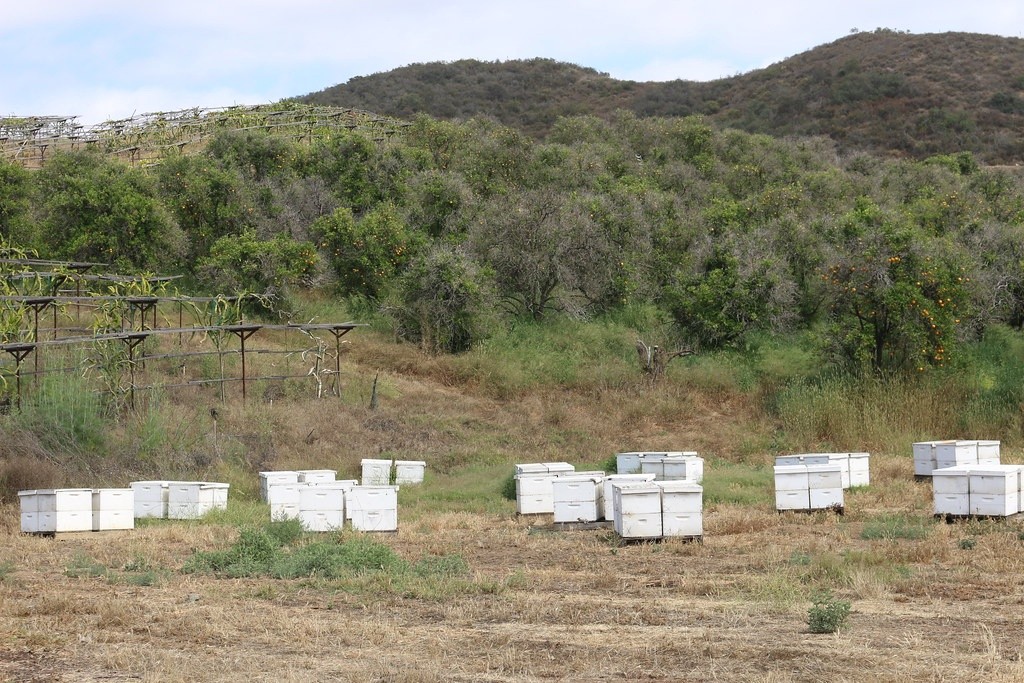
514;450;707;540
772;439;1024;517
17;480;230;533
258;459;426;533
